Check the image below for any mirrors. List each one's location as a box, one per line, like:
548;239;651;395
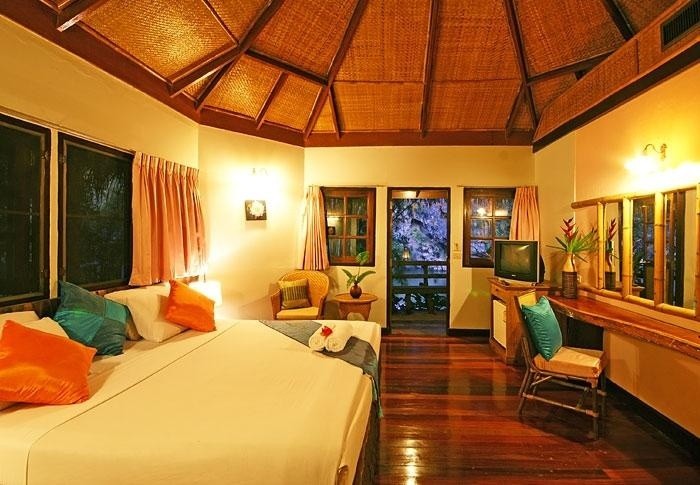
577;189;699;315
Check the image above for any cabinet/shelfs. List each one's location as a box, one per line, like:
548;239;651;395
487;277;554;362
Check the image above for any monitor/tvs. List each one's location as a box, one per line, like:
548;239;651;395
493;239;545;286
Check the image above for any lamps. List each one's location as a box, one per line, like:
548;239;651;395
402;247;411;260
624;143;667;171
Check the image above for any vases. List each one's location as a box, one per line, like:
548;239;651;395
606;250;617;291
562;251;576;298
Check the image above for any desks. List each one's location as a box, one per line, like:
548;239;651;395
334;293;378;322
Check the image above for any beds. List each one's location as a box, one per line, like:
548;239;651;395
0;283;381;485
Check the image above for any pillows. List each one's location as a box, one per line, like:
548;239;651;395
279;279;311;310
522;296;563;360
106;284;189;342
166;280;219;333
54;281;129;357
123;317;140;344
22;317;70;340
0;311;39;335
0;320;97;404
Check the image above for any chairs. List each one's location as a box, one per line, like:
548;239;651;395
514;287;611;439
271;269;330;320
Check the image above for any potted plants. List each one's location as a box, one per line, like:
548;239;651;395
342;252;376;298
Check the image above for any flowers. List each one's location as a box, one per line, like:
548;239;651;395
550;218;598;254
592;219;620;250
